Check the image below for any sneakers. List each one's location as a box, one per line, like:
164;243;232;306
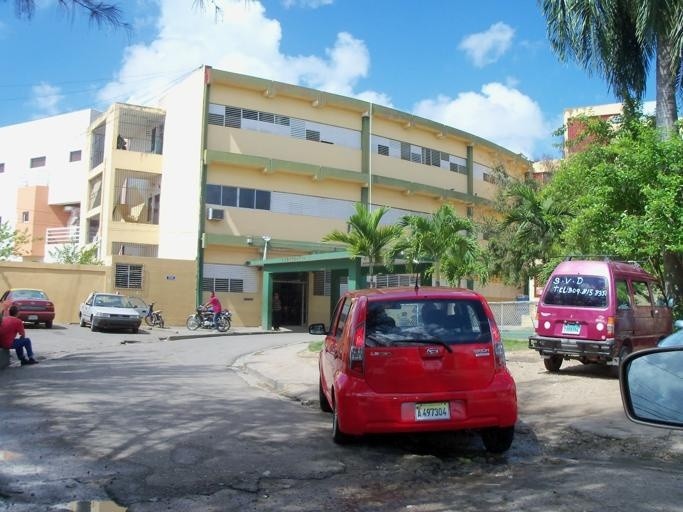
28;358;39;365
21;358;29;366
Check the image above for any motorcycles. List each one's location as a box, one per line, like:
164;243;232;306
144;303;164;328
185;306;232;333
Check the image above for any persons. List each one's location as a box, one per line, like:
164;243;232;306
203;291;223;329
270;291;282;331
0;306;40;366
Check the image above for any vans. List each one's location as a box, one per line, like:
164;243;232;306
524;251;675;378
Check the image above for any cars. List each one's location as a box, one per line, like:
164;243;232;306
75;290;147;333
655;319;683;349
0;287;56;329
308;271;521;453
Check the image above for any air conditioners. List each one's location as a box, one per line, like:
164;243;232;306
207;207;225;222
